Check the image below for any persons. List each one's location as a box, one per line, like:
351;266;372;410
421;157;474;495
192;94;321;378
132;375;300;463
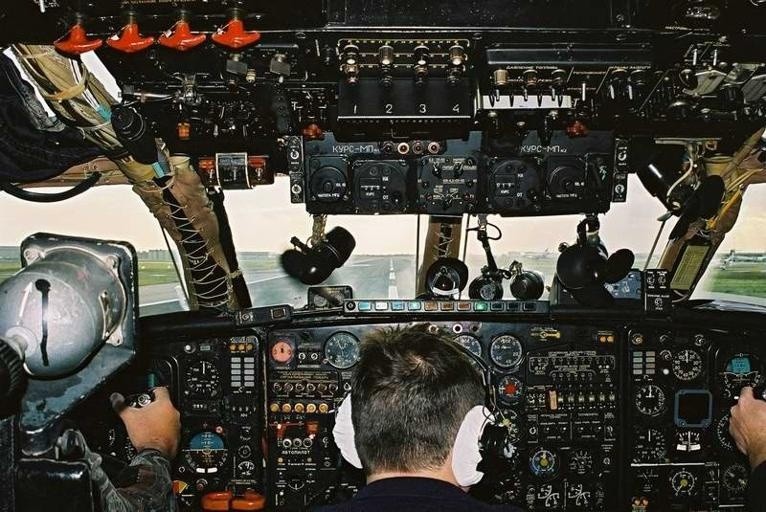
729;385;766;511
52;386;184;512
309;327;512;511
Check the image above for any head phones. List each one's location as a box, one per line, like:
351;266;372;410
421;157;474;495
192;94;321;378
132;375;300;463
326;325;512;487
